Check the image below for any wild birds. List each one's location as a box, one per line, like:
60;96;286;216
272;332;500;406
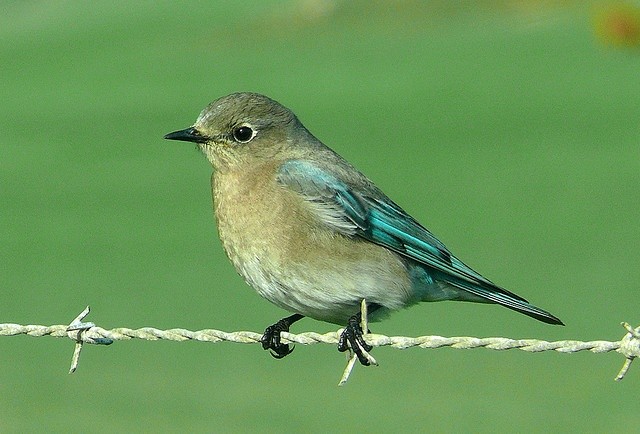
161;89;567;369
